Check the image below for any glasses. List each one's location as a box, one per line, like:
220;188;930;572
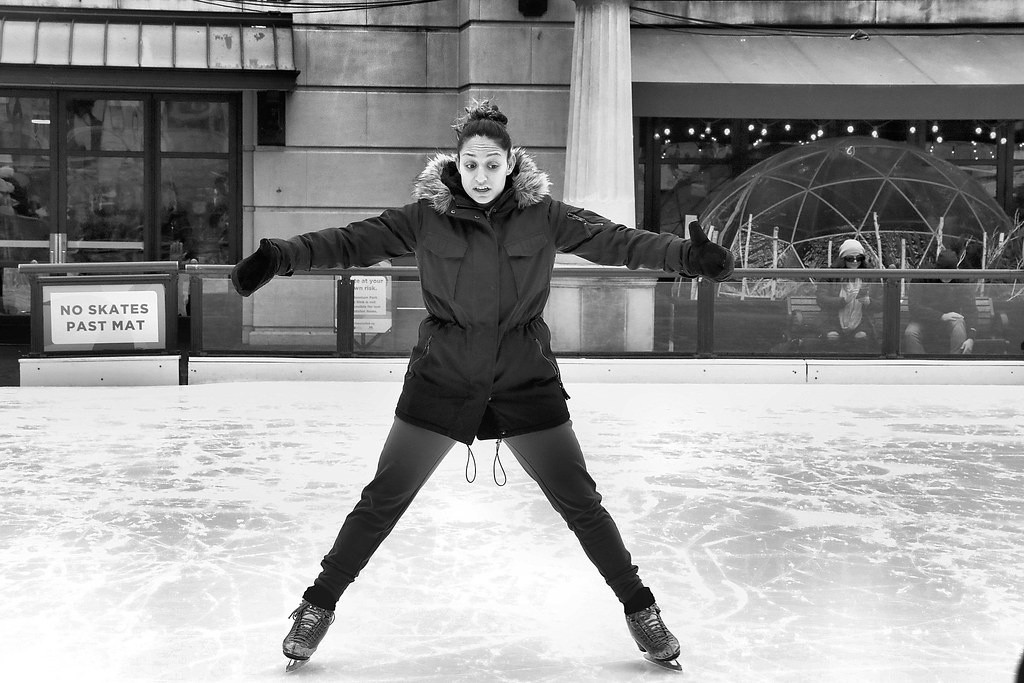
844;255;864;262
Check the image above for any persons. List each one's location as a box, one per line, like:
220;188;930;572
903;247;979;358
816;239;885;354
159;175;228;270
226;101;735;663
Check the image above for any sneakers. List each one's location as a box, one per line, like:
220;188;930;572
624;586;684;671
282;598;335;673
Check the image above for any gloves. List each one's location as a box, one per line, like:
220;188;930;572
231;237;282;298
688;222;734;283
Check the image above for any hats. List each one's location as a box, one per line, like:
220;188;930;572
937;250;958;269
839;239;865;257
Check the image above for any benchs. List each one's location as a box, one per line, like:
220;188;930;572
784;295;1010;355
